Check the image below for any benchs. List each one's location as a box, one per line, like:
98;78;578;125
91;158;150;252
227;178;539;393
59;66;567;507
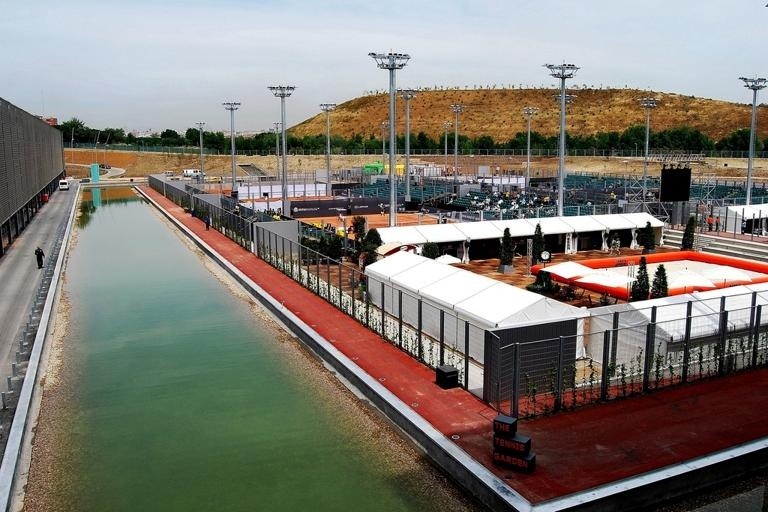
349;174;768;220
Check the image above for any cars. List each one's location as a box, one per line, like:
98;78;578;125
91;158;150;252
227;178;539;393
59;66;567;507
161;171;173;176
183;168;207;177
59;179;70;191
99;163;111;168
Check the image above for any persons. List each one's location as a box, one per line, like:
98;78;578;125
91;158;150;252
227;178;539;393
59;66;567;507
338;212;343;222
611;191;616;203
741;220;746;235
707;215;713;232
380;201;386;217
204;216;210;231
446;191;558;220
700;218;706;232
34;245;46;269
615;237;621;255
715;217;719;232
347;203;351;216
608;237;617;255
419;204;430;216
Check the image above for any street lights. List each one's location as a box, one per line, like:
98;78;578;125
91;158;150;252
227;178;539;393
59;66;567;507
451;105;464;183
368;52;415;227
738;74;768;203
522;107;539;177
223;101;241;192
443;120;452;177
194;121;206;173
320;103;337;184
638;96;657;161
267;84;297;215
541;61;581;216
